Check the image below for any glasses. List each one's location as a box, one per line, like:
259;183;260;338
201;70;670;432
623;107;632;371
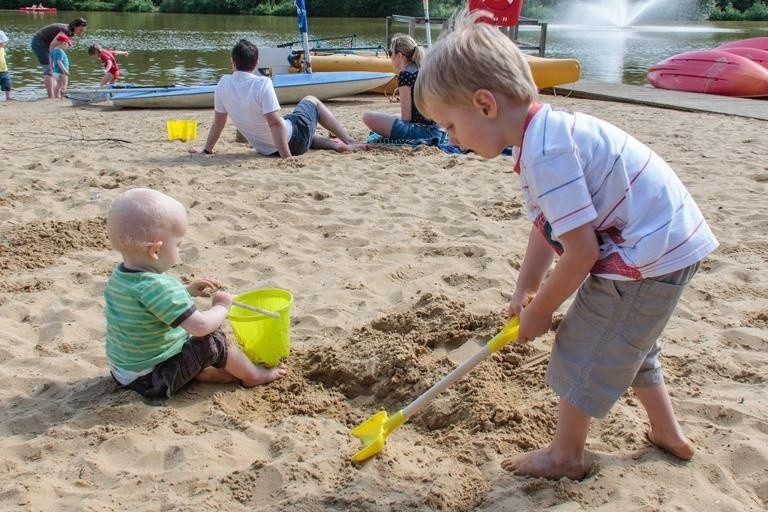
388;50;392;55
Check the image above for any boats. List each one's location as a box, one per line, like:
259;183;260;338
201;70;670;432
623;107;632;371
19;10;57;16
109;71;397;111
646;32;768;98
57;80;210;103
281;45;580;96
20;6;57;11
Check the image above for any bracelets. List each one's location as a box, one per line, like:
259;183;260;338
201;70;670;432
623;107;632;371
203;149;212;155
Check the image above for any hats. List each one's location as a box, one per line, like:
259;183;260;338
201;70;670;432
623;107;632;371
58;35;73;47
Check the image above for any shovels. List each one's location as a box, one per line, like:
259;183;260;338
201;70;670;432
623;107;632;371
349;314;520;463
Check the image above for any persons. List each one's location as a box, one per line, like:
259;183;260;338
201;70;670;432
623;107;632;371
102;185;288;398
31;17;88;99
413;21;718;482
88;43;129;86
0;30;18;100
188;39;371;159
361;32;448;145
50;34;72;100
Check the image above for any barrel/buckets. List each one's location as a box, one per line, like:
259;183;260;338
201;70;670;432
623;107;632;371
224;288;293;368
